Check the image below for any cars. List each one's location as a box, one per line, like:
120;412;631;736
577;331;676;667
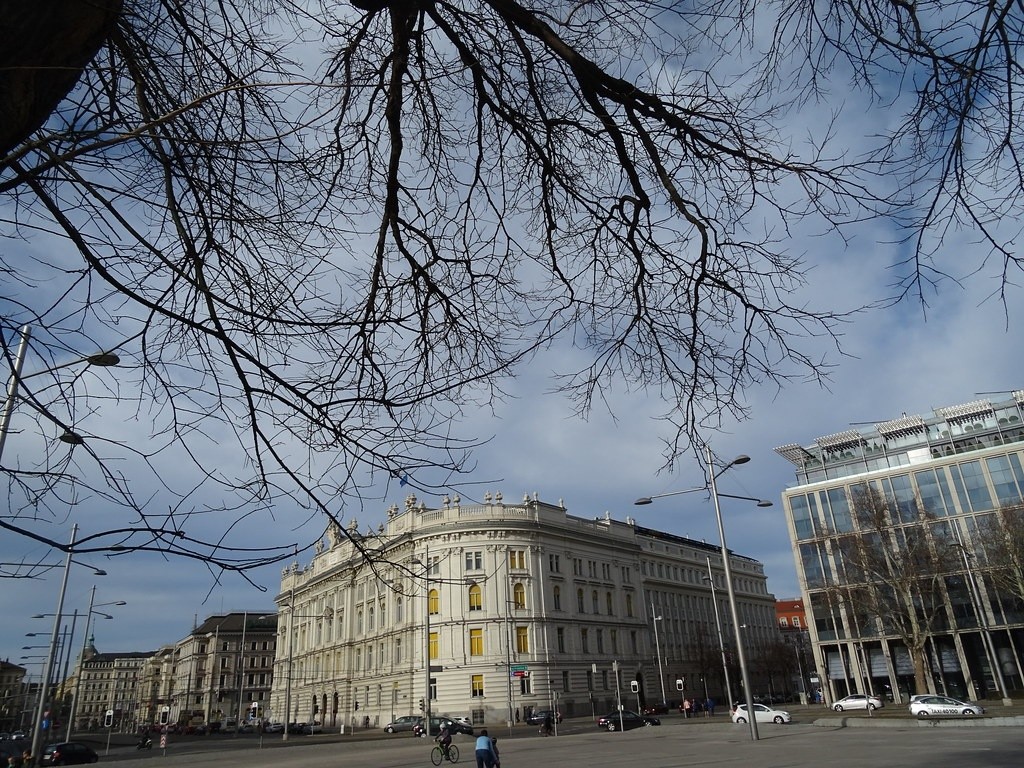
139;717;323;734
39;741;99;765
830;693;883;712
752;691;801;704
1;730;25;741
599;710;661;732
909;694;985;714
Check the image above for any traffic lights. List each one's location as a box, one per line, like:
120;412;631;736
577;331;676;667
104;709;114;728
160;707;170;725
676;679;684;691
250;702;258;720
355;701;359;711
631;681;639;693
419;696;424;711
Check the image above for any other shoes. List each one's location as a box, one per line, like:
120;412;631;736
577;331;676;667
445;755;450;761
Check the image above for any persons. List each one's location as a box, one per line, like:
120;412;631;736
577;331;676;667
433;724;452;759
691;699;699;717
685;698;691;718
516;708;520;722
476;730;500;768
807;691;815;704
539;713;554;736
704;698;714;718
143;733;149;745
172;722;203;736
7;749;35;768
366;716;370;727
527;709;532;716
609;705;616;712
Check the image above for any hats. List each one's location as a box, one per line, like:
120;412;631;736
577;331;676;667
440;724;446;728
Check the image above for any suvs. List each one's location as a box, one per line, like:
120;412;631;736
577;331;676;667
384;715;474;736
645;703;669;716
526;710;563;725
731;703;792;724
679;697;707;712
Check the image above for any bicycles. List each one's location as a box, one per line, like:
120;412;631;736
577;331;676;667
431;739;460;766
539;722;559;737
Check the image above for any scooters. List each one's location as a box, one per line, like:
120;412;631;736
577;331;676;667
136;738;153;751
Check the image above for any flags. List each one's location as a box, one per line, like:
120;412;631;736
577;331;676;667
400;475;407;487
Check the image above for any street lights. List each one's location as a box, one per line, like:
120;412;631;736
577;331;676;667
702;556;735;715
634;445;773;741
0;325;121;463
383;545;476;736
651;603;667;704
17;523;126;762
503;575;525;727
259;587;333;740
